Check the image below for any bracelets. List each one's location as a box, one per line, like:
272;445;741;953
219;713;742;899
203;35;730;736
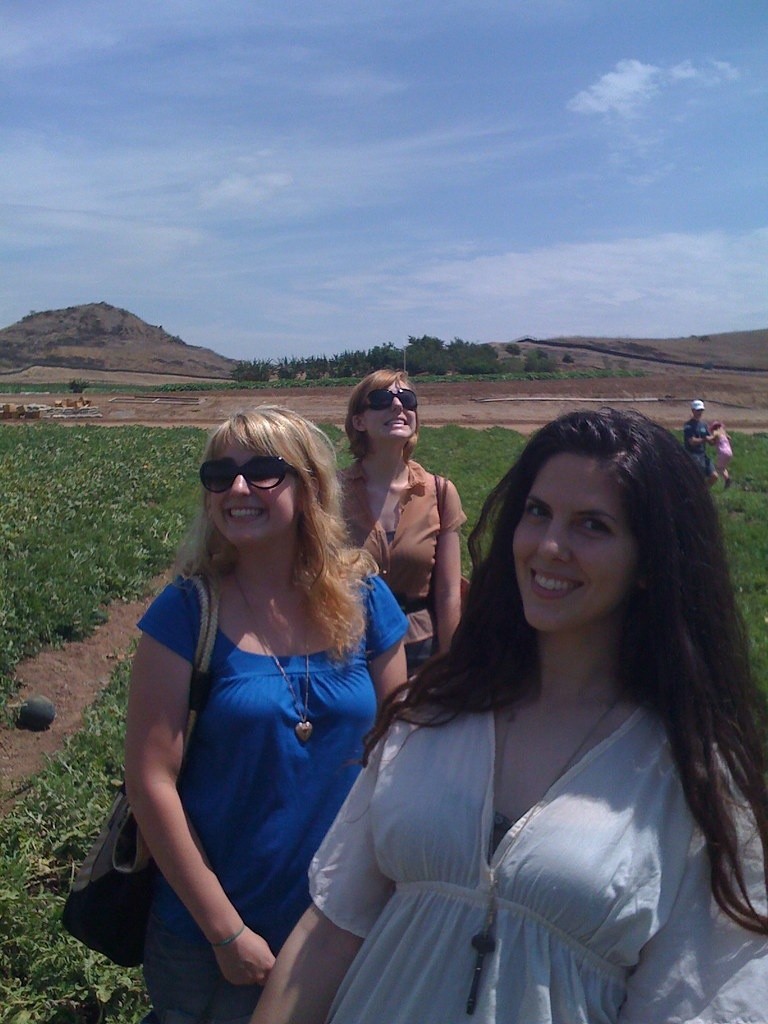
209;922;245;946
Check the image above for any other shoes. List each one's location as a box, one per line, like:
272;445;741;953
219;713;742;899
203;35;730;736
724;479;731;489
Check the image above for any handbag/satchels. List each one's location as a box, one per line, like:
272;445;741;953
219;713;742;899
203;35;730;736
62;573;219;967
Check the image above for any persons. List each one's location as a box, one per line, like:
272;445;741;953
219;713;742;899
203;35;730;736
682;399;719;494
124;368;768;1024
706;422;733;490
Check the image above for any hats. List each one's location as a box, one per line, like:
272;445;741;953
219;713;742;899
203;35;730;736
710;422;725;432
691;400;704;410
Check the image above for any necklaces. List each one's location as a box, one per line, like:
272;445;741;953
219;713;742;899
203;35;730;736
464;675;633;1015
232;564;318;743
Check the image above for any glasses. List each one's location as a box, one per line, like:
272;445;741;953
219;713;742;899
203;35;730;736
200;455;314;493
360;388;417;411
